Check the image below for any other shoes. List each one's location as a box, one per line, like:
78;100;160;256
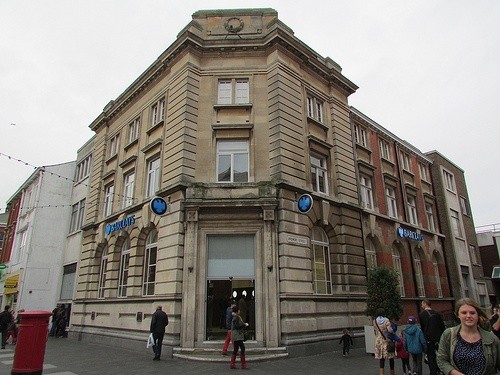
222;352;229;356
407;369;412;375
153;353;161;361
2;346;5;349
413;371;417;375
343;354;349;357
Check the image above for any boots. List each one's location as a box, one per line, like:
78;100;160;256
379;368;384;375
240;355;249;369
230;354;238;369
390;369;394;375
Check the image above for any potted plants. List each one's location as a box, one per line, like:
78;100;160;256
363;265;402;355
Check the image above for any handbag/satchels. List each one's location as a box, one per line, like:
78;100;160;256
243;330;248;342
146;333;155;349
387;341;395;352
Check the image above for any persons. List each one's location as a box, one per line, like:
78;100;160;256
487;301;500;340
49;304;68;338
0;305;12;349
6;309;24;344
419;299;445;375
396;330;411;375
229;306;250;370
403;316;426;375
339;329;354;358
436;297;500;375
150;305;169;360
222;301;239;356
373;309;397;375
